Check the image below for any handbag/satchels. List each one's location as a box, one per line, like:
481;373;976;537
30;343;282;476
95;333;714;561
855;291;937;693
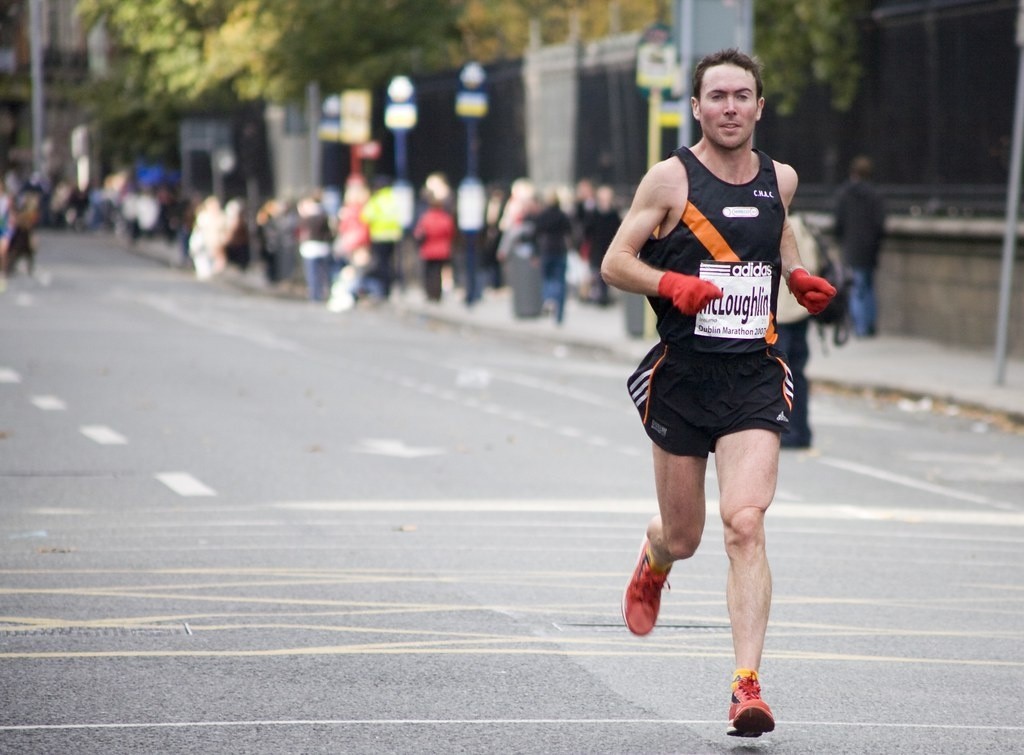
805;217;848;324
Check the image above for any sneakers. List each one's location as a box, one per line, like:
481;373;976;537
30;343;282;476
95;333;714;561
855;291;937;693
726;673;776;738
621;539;673;635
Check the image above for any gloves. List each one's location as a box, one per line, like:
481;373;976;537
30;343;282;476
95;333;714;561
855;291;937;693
785;268;836;315
658;272;724;316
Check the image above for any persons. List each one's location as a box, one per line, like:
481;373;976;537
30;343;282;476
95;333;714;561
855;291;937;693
829;150;886;338
600;50;836;737
0;169;180;278
414;167;623;324
184;174;404;307
